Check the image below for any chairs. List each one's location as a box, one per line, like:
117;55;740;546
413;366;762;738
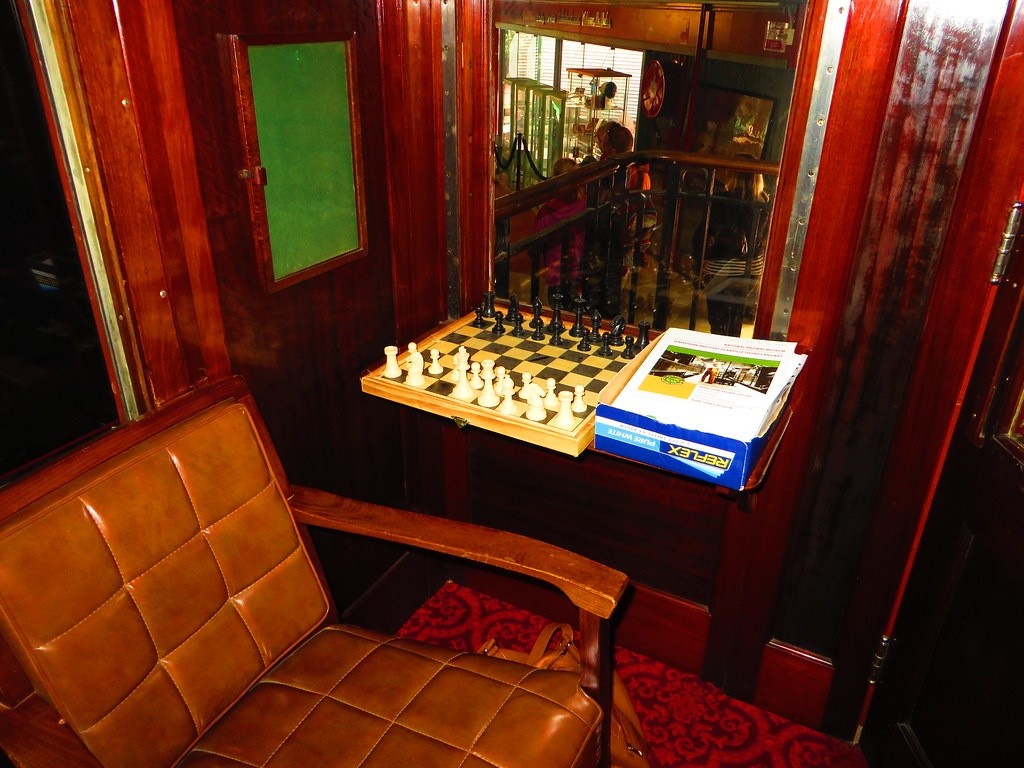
1;376;628;767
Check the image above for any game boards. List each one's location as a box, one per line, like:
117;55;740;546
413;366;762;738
359;304;653;458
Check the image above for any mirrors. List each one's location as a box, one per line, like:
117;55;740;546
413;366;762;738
480;0;818;342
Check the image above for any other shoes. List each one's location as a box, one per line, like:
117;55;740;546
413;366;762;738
640;251;650;267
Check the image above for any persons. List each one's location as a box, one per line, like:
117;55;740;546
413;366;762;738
494;120;772;337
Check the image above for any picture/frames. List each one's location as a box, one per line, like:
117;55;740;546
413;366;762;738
680;79;778;196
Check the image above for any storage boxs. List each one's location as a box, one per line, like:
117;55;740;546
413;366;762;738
593;330;792;491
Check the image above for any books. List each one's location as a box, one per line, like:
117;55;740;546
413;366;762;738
609;326;808;443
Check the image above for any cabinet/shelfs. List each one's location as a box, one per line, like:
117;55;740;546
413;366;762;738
565;68;632;165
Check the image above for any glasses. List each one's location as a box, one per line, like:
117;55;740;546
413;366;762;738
606;124;618;149
